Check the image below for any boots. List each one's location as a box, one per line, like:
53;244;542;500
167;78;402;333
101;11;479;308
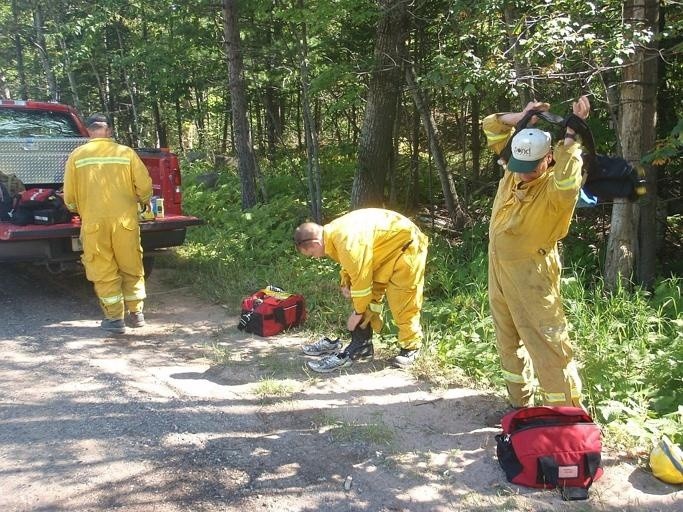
343;327;372;362
392;349;419;369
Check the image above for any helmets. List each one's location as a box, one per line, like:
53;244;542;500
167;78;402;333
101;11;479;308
648;436;682;484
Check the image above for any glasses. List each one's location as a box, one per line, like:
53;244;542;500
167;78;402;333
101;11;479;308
294;239;312;245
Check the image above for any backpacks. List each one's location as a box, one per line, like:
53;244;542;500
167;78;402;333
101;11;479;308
0;170;27;220
10;188;70;226
502;110;635;208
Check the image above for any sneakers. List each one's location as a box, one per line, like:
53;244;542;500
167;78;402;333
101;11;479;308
100;320;124;334
303;337;339;356
127;312;144;327
307;352;351;373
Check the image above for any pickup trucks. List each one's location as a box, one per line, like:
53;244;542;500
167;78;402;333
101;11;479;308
0;98;209;285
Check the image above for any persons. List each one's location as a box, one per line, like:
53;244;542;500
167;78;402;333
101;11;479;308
483;95;592;413
294;208;429;368
63;112;155;333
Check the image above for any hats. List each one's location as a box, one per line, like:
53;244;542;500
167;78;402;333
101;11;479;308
86;113;110;127
506;128;550;172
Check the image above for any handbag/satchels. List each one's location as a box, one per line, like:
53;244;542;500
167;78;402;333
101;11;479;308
241;286;305;337
495;406;603;490
